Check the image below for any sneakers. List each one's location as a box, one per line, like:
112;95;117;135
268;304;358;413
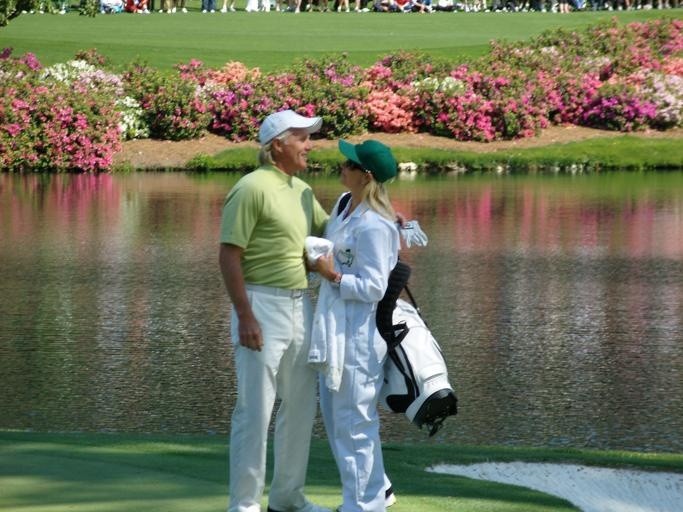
336;487;396;512
21;10;66;15
143;6;236;14
267;502;334;512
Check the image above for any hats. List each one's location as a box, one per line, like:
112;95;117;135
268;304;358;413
339;140;397;183
259;110;323;146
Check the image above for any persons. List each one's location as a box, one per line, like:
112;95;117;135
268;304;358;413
303;141;399;512
220;109;407;512
98;0;683;15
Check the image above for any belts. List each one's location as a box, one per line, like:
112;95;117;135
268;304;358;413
245;284;305;300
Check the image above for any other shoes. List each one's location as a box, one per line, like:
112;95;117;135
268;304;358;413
277;3;671;14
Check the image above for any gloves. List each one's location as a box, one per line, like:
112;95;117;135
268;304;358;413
399;220;428;249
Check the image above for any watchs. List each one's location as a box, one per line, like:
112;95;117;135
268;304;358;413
329;271;342;289
